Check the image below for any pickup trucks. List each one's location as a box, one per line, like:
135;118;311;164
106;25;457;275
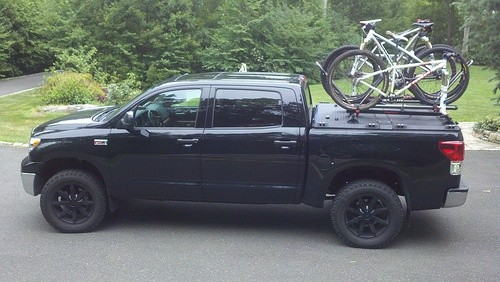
20;72;469;248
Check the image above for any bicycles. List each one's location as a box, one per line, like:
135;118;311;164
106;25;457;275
321;19;470;112
